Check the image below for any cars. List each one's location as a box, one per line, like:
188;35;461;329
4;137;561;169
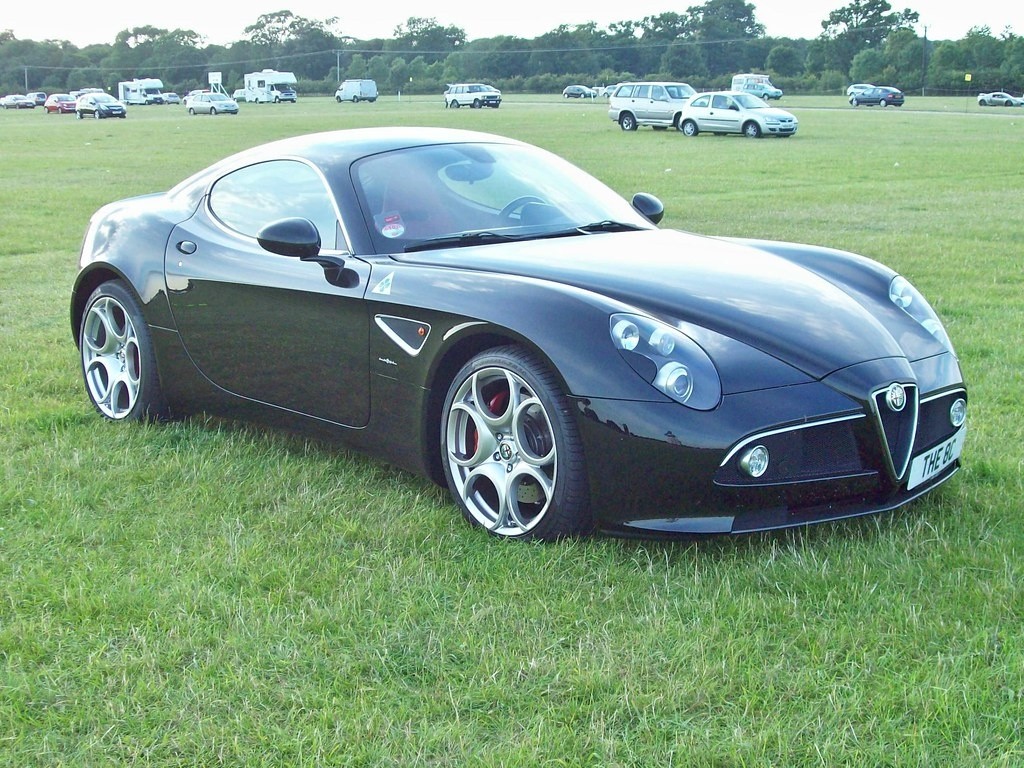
847;84;876;96
185;93;238;115
1;95;36;109
848;86;905;107
600;85;622;98
74;93;126;119
163;92;180;105
43;93;76;113
562;85;598;97
182;90;210;107
679;91;798;138
744;83;783;100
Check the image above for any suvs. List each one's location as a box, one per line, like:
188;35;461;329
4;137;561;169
444;83;501;108
608;83;698;131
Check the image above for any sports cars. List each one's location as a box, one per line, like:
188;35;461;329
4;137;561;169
70;125;969;543
977;92;1024;106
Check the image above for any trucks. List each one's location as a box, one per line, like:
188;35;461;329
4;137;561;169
118;78;164;105
244;69;297;103
731;74;775;92
68;88;106;97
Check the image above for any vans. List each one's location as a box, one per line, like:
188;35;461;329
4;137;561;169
233;88;245;102
26;91;47;105
334;78;378;102
